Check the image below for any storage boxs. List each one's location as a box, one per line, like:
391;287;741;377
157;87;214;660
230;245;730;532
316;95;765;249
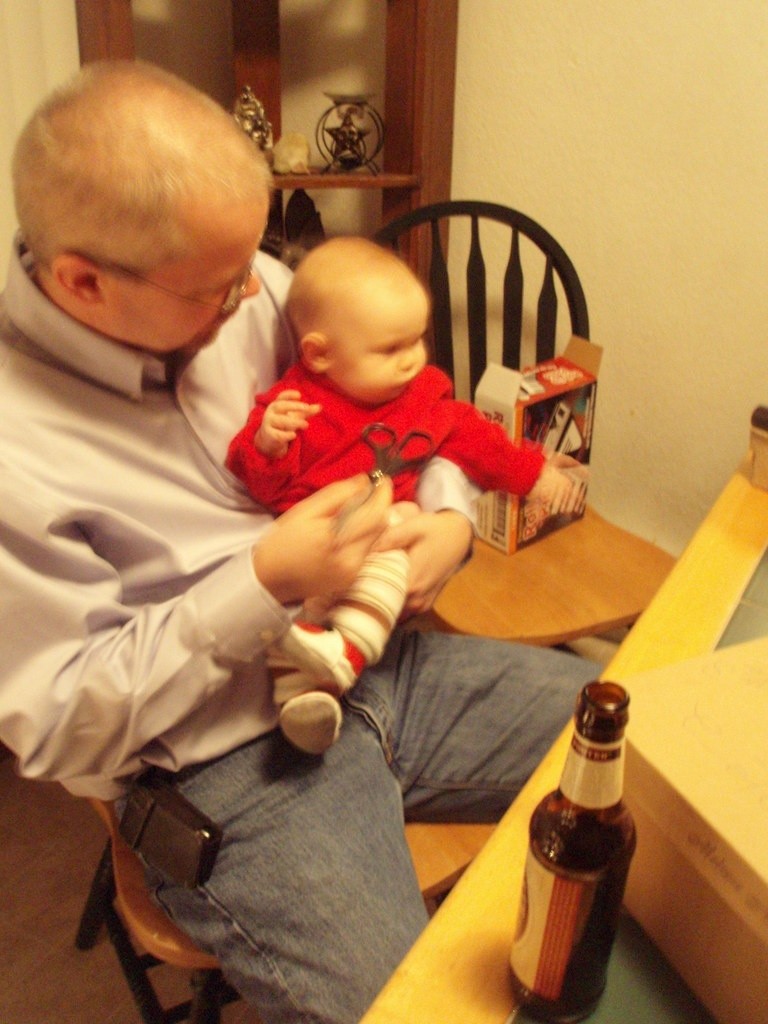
608;636;768;1024
469;339;603;558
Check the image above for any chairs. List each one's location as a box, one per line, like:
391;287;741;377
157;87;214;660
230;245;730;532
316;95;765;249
371;198;680;646
76;771;496;1024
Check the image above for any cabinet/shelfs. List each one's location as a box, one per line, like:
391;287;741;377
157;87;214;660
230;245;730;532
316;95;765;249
75;1;457;366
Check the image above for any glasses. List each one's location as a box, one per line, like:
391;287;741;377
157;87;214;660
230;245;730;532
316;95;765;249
50;246;255;318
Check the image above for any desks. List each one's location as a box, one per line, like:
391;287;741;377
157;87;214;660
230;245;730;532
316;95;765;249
364;472;768;1024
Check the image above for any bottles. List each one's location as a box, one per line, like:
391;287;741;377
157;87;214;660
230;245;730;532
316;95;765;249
510;679;638;1024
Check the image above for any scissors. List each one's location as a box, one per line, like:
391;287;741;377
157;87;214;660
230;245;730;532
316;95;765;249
362;421;434;486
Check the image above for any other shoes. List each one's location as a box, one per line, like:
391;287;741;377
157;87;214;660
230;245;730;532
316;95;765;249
272;621;368;699
277;689;342;756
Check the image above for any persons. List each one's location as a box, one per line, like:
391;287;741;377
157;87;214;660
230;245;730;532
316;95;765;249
223;236;563;755
231;84;274;149
0;60;606;1024
